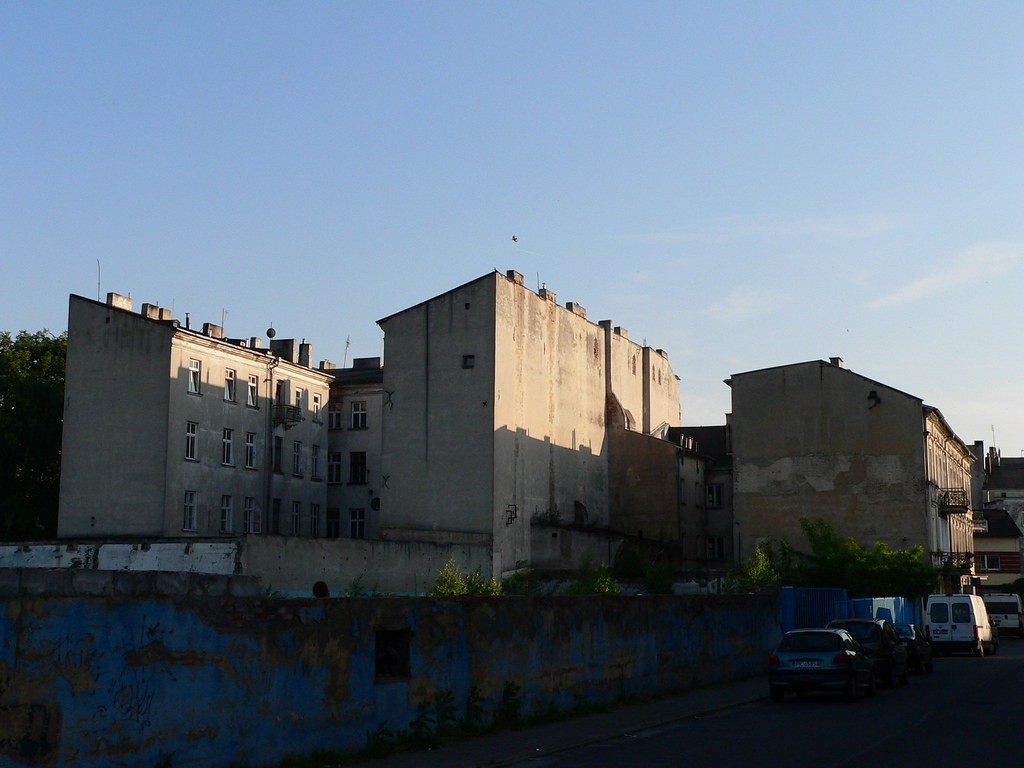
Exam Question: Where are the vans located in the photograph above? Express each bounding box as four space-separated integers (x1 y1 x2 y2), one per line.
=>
983 593 1024 640
925 593 999 656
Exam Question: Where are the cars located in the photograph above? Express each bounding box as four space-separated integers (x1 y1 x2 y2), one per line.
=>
893 624 933 676
766 628 874 703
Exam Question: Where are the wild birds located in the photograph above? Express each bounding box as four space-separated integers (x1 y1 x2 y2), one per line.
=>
512 234 518 242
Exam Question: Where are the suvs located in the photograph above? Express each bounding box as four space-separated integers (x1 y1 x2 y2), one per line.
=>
824 618 911 689
